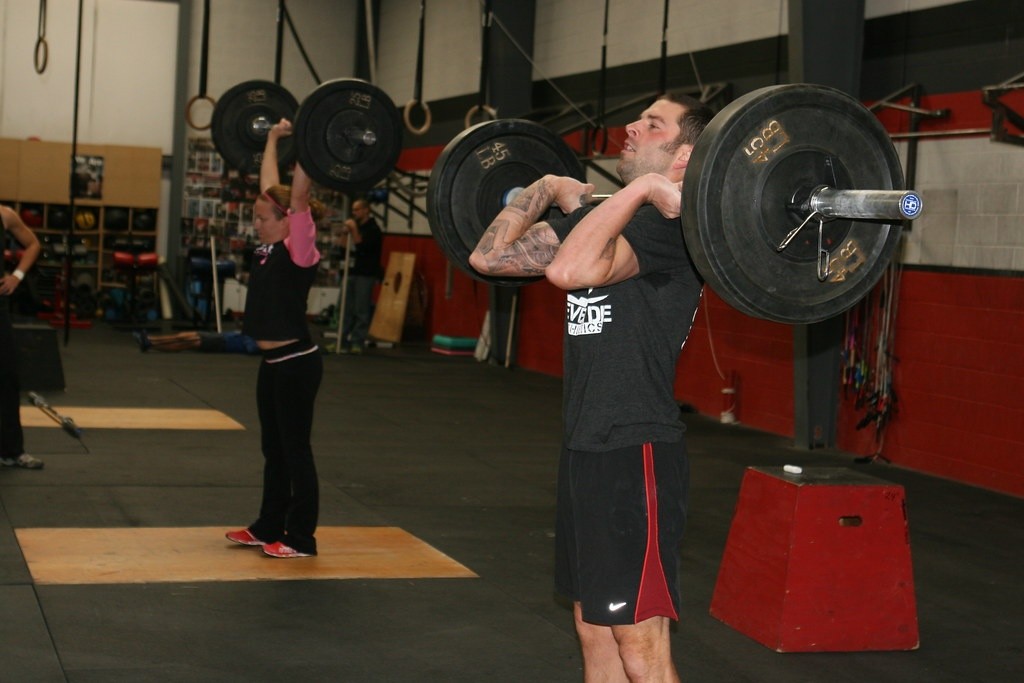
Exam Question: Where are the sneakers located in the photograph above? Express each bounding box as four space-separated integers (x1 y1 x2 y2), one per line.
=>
226 529 267 547
263 541 310 557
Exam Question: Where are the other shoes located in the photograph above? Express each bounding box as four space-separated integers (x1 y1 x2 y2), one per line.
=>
1 453 44 468
323 341 350 354
351 339 362 355
140 329 149 351
133 332 141 345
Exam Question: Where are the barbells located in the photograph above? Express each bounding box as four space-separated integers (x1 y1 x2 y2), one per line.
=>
210 78 405 193
425 82 924 326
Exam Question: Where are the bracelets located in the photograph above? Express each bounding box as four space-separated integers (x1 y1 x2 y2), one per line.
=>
13 270 23 279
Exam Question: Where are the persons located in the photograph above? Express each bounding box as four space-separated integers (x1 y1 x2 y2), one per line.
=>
337 200 384 346
470 91 715 683
0 206 43 469
132 330 260 354
225 119 323 557
